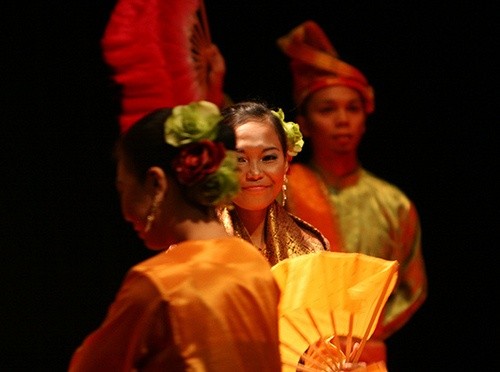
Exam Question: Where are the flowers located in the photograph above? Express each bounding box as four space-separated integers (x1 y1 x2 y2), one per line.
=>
165 100 241 207
270 108 305 175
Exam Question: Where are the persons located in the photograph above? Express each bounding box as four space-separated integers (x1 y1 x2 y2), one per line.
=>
204 22 426 372
214 102 368 372
68 98 283 372
